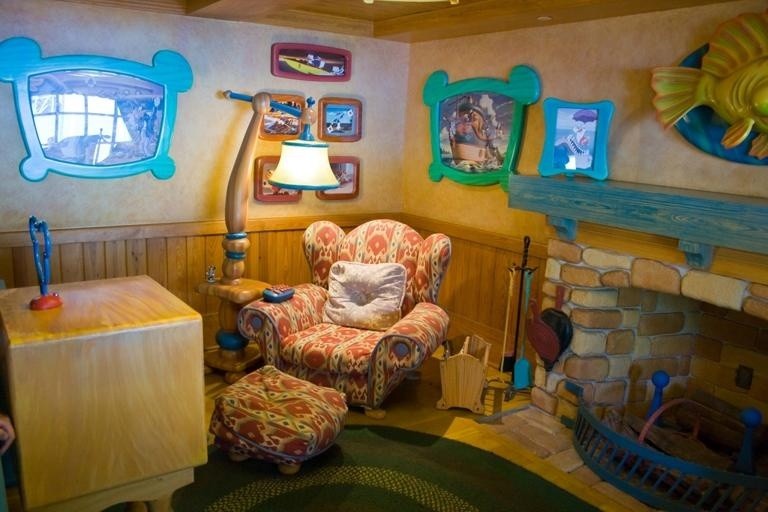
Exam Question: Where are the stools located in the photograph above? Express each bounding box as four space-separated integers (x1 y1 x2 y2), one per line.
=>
211 365 348 473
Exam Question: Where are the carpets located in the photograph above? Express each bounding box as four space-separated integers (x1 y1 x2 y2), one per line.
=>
171 424 603 510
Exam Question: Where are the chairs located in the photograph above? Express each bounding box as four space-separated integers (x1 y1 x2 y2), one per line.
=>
236 217 454 420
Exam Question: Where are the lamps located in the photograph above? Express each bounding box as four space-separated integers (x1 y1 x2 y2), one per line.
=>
194 91 342 385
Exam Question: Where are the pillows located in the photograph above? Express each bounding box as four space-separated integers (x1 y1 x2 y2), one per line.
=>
321 261 408 330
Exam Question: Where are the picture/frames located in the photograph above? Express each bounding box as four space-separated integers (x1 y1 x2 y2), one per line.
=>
537 95 616 182
317 96 363 143
258 93 306 140
272 41 352 82
421 65 542 192
317 155 360 201
254 155 303 203
0 36 194 182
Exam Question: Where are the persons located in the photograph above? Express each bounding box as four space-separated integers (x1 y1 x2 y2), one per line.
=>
0 413 17 453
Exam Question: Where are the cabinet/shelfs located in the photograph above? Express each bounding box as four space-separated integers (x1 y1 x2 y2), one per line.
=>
4 274 208 512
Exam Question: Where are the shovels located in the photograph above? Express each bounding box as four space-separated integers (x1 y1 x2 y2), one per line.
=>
513 269 532 391
